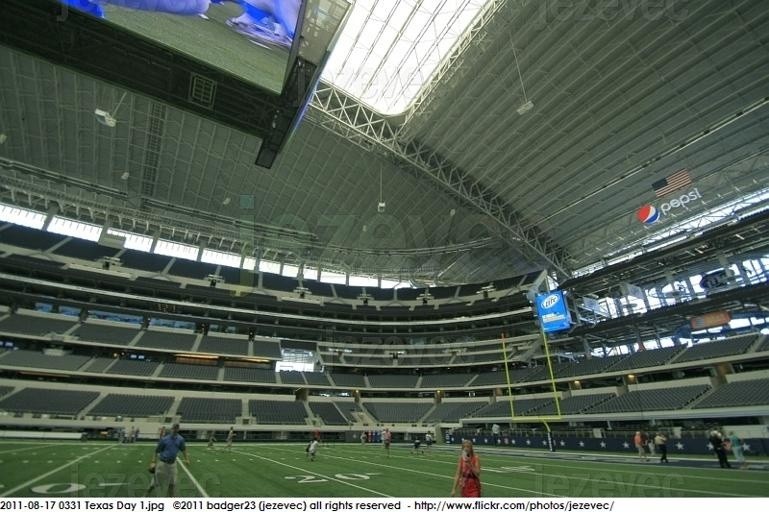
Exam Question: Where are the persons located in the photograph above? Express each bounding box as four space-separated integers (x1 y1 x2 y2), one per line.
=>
118 423 769 497
